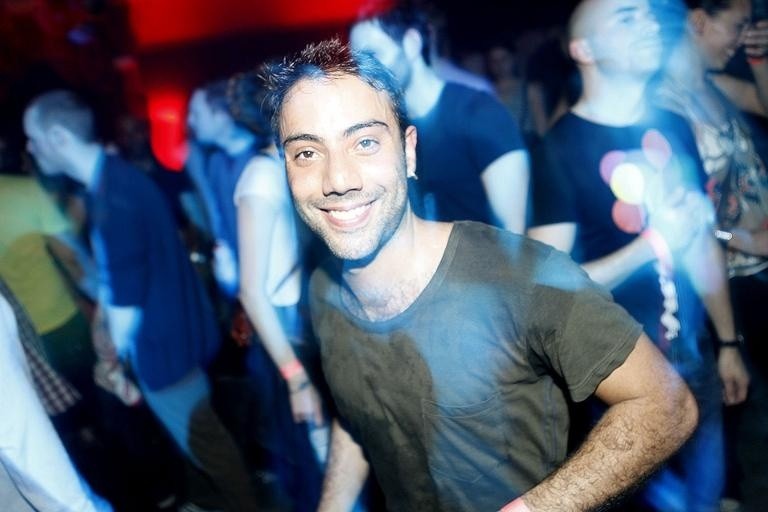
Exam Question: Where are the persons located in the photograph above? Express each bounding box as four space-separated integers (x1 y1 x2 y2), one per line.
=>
0 0 767 512
